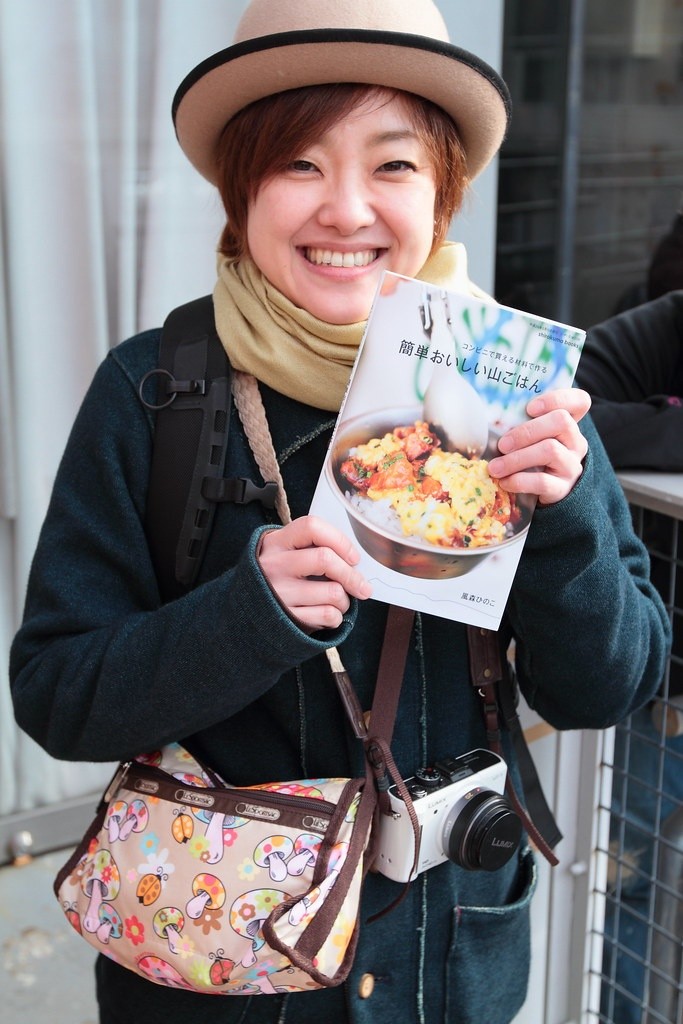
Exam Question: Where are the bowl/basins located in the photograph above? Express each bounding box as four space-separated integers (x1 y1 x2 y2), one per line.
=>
323 407 535 580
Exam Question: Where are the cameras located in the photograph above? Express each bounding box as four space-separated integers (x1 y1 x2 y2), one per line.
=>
374 749 523 883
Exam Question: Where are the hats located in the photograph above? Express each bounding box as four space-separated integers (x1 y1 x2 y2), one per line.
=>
173 0 510 185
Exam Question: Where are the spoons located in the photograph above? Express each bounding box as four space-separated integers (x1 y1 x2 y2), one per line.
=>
422 287 489 458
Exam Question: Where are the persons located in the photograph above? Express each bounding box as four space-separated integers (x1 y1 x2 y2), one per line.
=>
576 290 683 1024
10 0 669 1024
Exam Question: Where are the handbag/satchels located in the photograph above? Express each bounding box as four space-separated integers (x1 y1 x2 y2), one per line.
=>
53 745 376 994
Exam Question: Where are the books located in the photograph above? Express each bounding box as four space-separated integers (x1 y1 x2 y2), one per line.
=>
307 270 587 631
